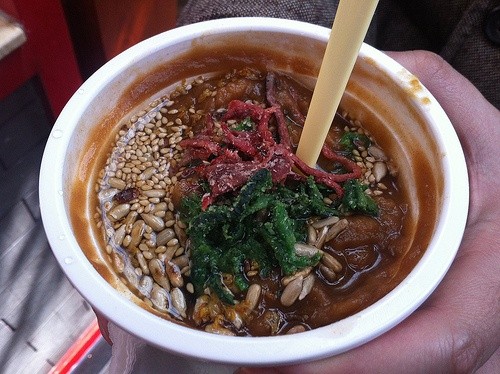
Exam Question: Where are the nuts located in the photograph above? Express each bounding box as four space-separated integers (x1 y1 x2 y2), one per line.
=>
94 91 399 337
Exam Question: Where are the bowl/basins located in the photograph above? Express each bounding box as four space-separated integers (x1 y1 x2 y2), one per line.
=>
38 14 472 367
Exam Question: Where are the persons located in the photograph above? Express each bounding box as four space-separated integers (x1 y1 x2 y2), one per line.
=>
86 41 500 373
